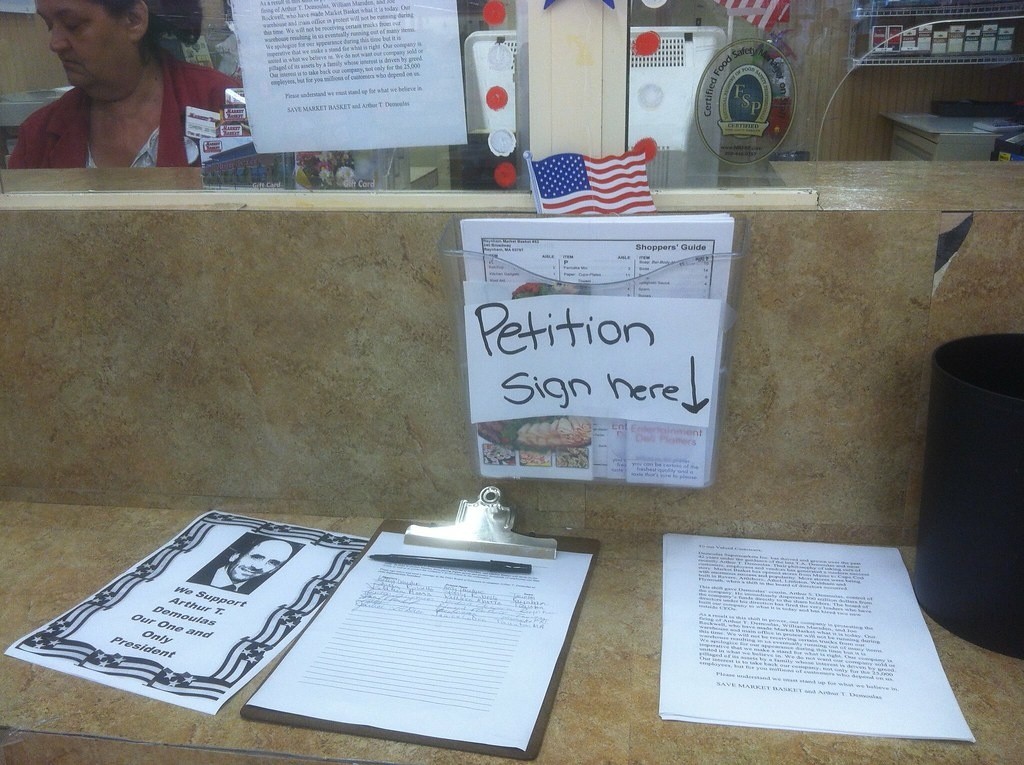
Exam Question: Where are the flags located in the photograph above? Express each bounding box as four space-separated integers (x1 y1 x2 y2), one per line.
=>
531 148 658 215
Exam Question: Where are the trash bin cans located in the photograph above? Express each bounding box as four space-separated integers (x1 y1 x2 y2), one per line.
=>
902 333 1024 664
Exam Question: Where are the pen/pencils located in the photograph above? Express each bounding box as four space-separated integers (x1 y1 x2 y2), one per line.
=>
368 553 532 573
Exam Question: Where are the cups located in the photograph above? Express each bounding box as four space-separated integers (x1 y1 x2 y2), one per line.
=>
913 333 1024 659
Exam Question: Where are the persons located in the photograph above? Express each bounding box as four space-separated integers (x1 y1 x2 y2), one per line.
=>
190 540 294 595
7 0 254 169
